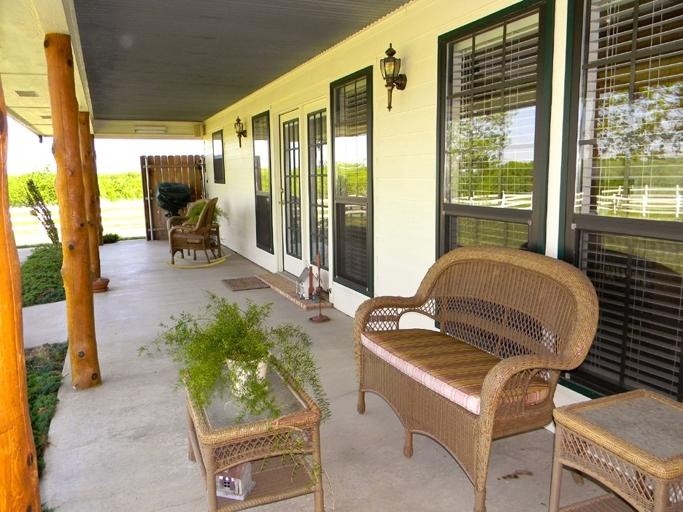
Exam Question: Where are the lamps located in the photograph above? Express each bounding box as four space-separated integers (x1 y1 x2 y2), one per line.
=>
379 41 408 112
233 115 247 149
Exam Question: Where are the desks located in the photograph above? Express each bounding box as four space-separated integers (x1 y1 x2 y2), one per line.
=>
547 388 683 512
181 357 325 512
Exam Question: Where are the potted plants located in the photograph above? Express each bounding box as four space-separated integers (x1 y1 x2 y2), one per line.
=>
134 289 333 491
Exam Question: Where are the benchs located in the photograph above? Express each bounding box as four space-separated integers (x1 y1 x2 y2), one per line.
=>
353 245 600 512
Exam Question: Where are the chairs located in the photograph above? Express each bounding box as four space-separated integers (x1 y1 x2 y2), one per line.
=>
166 199 210 255
168 197 217 265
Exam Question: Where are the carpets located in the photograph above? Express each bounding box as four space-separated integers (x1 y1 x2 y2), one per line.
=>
220 276 271 291
